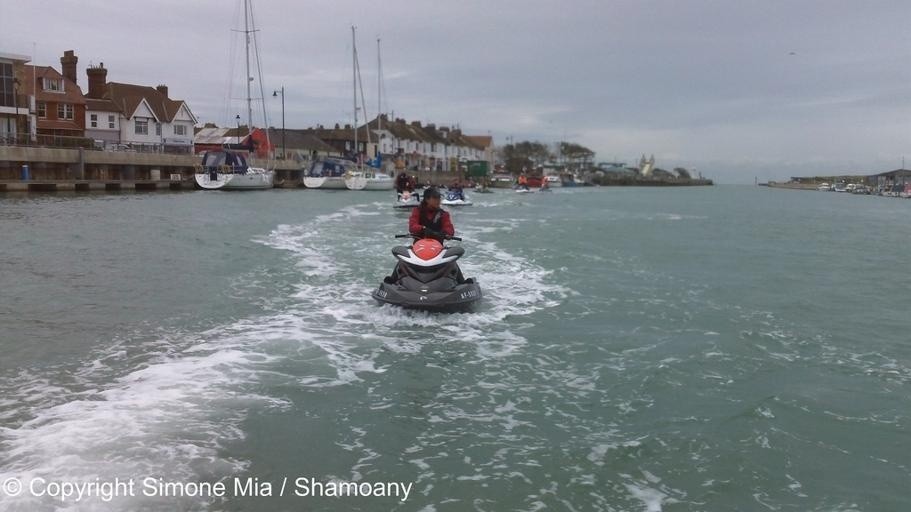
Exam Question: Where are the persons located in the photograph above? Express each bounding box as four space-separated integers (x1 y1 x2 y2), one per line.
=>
393 173 416 200
449 182 464 200
541 177 549 189
518 173 528 185
408 186 455 247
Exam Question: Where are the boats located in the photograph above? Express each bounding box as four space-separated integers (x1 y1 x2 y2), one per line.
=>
422 183 448 194
473 184 552 195
394 191 424 207
372 231 481 308
441 190 472 206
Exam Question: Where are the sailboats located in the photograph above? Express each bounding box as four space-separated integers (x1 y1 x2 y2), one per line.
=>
194 1 279 190
303 23 376 188
344 39 396 191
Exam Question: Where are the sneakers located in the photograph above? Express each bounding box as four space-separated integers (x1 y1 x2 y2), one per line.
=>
384 276 398 284
462 278 474 284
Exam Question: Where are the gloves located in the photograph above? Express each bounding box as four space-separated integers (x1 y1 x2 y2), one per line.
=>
424 227 434 236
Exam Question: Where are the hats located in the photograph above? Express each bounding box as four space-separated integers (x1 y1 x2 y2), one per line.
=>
424 187 446 199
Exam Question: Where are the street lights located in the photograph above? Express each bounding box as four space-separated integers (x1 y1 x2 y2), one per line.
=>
236 115 241 149
12 77 22 145
